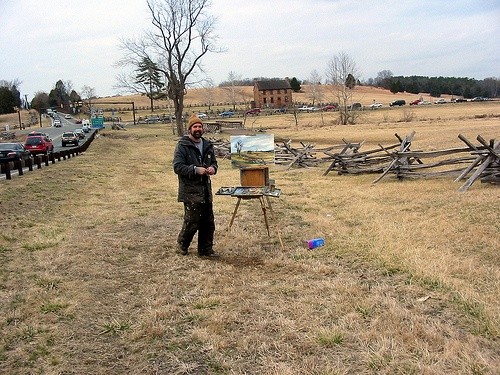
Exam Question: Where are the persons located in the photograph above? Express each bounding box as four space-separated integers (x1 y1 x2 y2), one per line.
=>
172 113 220 258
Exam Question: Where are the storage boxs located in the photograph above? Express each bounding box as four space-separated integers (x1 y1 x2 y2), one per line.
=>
239 167 269 187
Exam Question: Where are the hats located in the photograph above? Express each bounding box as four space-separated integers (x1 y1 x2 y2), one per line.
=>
187 114 203 130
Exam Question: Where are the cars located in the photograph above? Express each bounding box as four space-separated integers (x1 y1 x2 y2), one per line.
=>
0 142 34 164
145 111 209 124
297 102 385 113
219 110 235 118
73 128 85 139
45 108 62 128
75 118 92 133
64 113 72 119
409 97 485 106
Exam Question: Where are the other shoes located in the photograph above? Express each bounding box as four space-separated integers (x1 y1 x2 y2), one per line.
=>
180 244 188 255
203 252 220 258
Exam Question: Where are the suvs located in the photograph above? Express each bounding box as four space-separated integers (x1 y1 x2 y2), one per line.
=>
245 107 262 115
22 131 54 157
61 131 79 147
389 99 406 107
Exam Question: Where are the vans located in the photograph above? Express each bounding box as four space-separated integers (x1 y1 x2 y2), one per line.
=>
274 109 287 114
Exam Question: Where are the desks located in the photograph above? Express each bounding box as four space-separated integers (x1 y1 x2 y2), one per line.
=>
215 184 283 239
